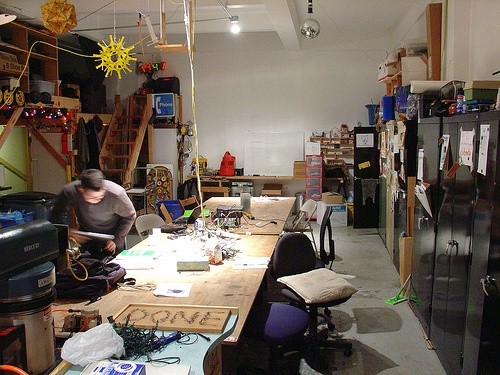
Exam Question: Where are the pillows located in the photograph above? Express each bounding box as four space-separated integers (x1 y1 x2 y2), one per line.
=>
276 268 358 303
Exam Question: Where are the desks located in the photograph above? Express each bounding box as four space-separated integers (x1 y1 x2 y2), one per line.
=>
126 188 149 234
53 197 296 375
187 175 306 181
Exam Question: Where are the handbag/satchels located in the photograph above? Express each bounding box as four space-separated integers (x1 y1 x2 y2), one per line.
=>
55 258 126 299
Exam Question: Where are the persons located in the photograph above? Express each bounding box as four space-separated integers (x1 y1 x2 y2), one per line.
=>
52 169 136 262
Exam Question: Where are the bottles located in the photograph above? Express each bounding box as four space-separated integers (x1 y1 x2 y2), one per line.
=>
80 308 102 332
240 185 251 212
457 93 463 115
75 310 82 333
63 309 75 332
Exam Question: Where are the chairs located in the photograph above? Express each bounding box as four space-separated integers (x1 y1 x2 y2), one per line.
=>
283 199 318 256
179 195 199 211
273 233 352 371
238 301 309 375
135 213 165 241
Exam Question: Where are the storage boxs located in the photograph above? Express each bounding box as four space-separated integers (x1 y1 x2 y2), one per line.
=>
29 80 56 95
305 141 323 221
463 80 500 100
419 92 439 118
293 161 306 177
316 192 348 228
377 60 397 82
261 183 283 196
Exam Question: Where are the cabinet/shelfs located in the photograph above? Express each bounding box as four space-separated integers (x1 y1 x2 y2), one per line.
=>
0 22 58 106
351 110 500 375
